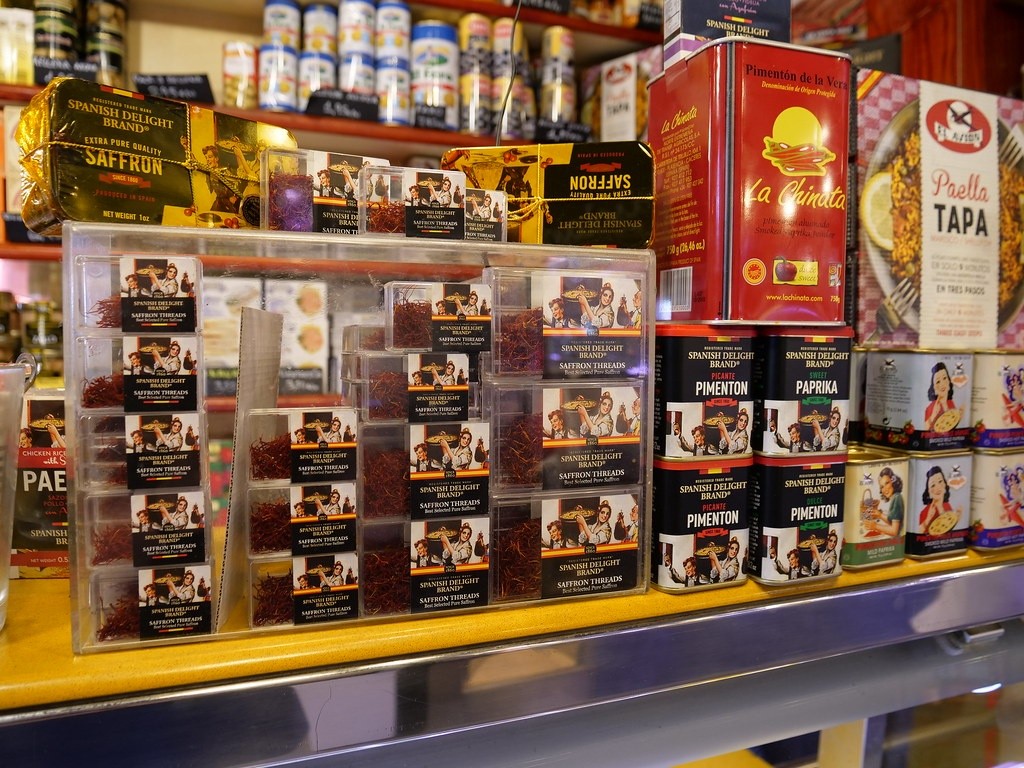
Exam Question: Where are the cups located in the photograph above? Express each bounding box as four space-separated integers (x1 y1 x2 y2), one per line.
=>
0 352 38 632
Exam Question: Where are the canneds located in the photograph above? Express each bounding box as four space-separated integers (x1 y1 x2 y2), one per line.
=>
32 0 129 93
651 323 1024 597
226 0 601 144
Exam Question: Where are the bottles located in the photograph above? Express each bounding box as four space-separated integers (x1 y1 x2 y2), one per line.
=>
0 0 35 87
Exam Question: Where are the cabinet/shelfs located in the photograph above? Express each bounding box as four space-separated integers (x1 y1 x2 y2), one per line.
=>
0 0 1024 768
